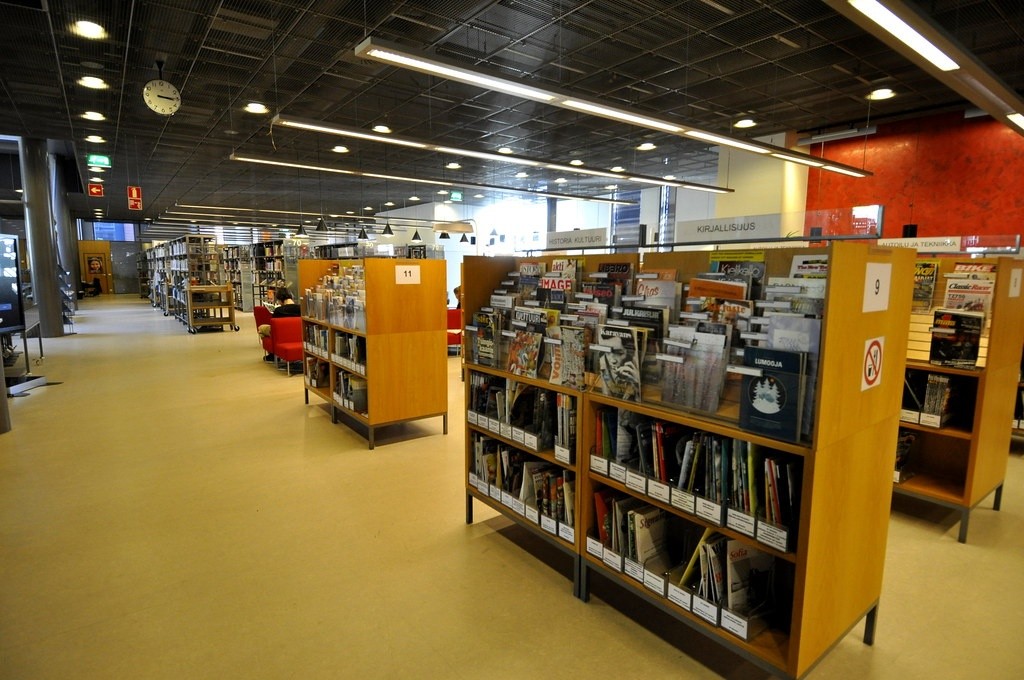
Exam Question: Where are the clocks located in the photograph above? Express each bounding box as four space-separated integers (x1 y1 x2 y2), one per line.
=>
143 80 181 115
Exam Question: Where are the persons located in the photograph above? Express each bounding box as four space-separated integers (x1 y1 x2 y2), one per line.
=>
88 257 103 274
262 286 302 363
598 328 639 403
453 285 461 310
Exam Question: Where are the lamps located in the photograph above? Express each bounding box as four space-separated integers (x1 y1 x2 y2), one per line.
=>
137 0 1024 248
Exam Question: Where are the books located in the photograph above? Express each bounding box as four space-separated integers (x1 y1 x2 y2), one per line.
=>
896 428 919 471
469 261 824 614
909 261 995 369
925 373 953 415
302 275 365 412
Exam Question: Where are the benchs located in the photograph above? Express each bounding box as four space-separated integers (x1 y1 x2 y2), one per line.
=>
447 309 461 357
254 304 303 376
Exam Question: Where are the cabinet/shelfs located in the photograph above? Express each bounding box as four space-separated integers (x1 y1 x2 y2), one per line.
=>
892 256 1024 543
455 239 918 680
297 255 450 450
142 234 444 336
